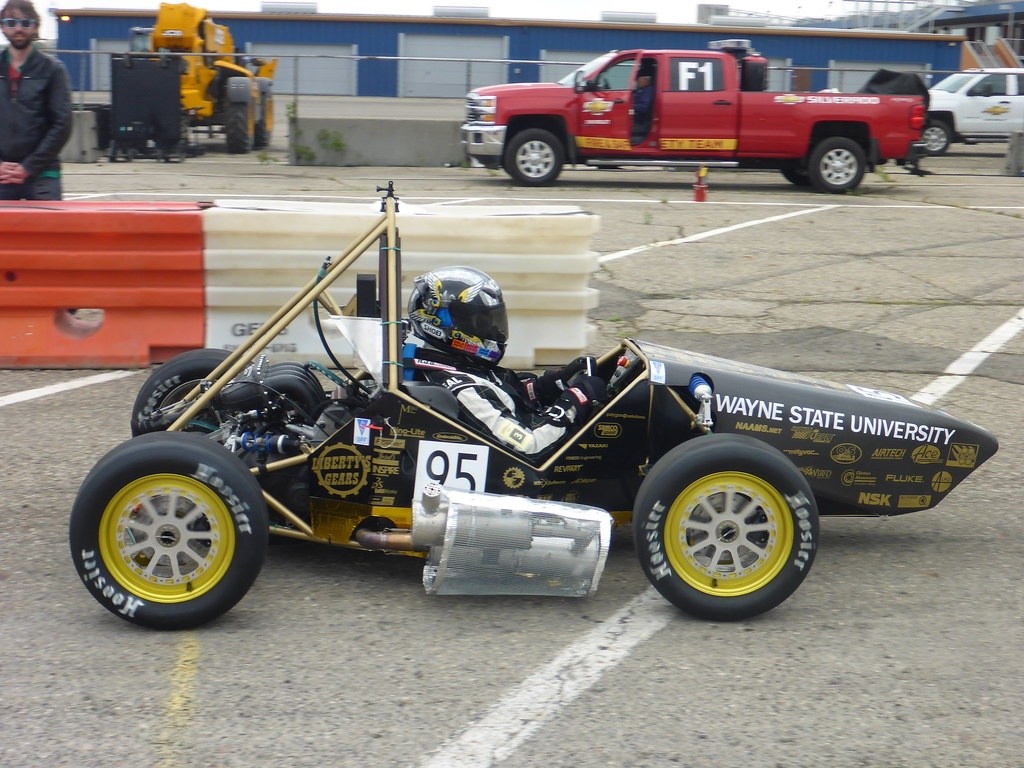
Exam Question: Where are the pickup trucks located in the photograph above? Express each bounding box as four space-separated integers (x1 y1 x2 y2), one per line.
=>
458 30 934 196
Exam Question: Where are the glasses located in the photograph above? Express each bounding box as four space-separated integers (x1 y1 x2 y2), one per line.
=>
0 18 35 29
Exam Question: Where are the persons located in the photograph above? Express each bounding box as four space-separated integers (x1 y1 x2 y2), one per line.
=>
632 64 656 124
408 266 606 466
0 0 76 316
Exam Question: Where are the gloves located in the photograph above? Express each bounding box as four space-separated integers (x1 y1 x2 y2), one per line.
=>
538 356 596 393
544 374 606 430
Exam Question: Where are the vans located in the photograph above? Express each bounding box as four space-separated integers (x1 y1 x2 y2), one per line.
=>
919 68 1024 158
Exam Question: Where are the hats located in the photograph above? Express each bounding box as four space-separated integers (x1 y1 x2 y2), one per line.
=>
636 66 654 80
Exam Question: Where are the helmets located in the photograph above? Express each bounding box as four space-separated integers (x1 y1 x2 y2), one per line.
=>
407 266 509 373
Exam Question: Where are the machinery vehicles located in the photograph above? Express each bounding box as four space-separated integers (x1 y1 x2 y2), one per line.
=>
125 4 278 153
